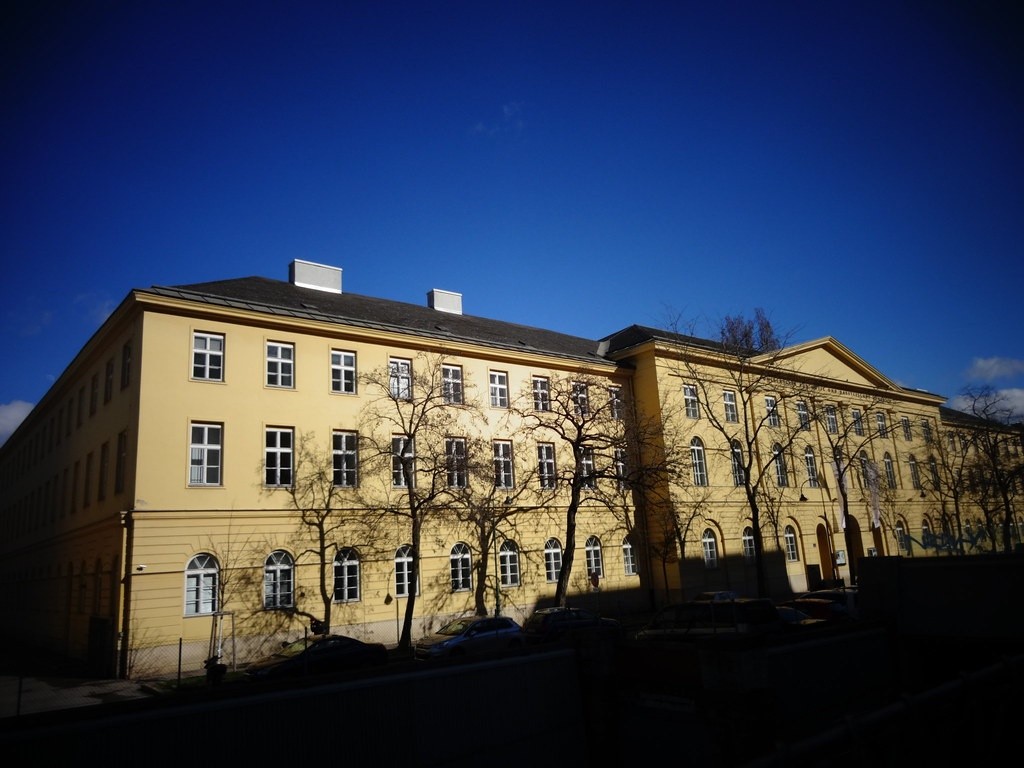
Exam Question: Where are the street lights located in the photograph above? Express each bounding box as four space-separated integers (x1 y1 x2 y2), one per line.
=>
920 479 952 555
491 479 515 618
799 479 840 584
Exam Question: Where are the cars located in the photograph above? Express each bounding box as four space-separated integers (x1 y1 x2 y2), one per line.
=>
630 586 863 659
522 606 624 656
414 614 530 667
241 634 389 690
